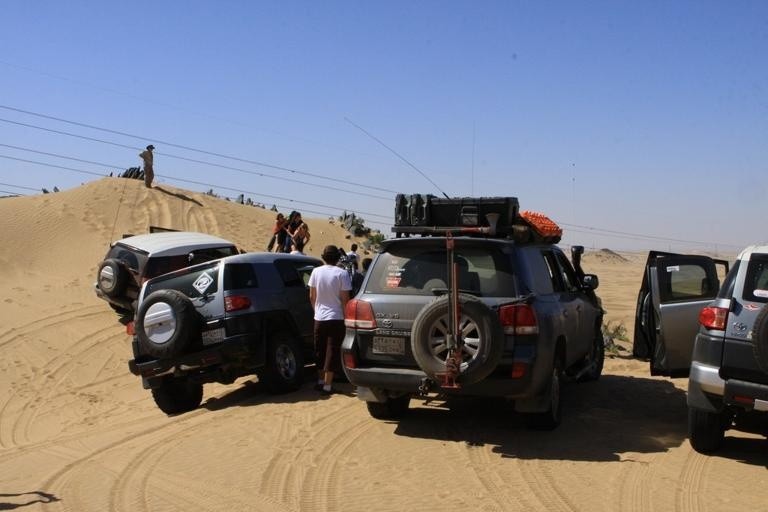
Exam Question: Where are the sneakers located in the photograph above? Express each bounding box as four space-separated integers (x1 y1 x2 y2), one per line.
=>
314 384 335 396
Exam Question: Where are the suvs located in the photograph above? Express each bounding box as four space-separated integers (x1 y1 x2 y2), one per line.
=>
125 250 364 414
340 192 607 429
632 242 768 453
94 226 238 313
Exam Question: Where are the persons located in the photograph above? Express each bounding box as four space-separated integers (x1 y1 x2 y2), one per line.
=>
336 243 373 294
139 144 155 188
307 246 353 397
267 211 310 253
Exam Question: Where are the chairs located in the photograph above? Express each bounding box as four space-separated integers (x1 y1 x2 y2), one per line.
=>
456 257 480 291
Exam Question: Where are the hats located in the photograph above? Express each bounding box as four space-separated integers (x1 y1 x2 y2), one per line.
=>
146 145 154 149
325 245 338 256
